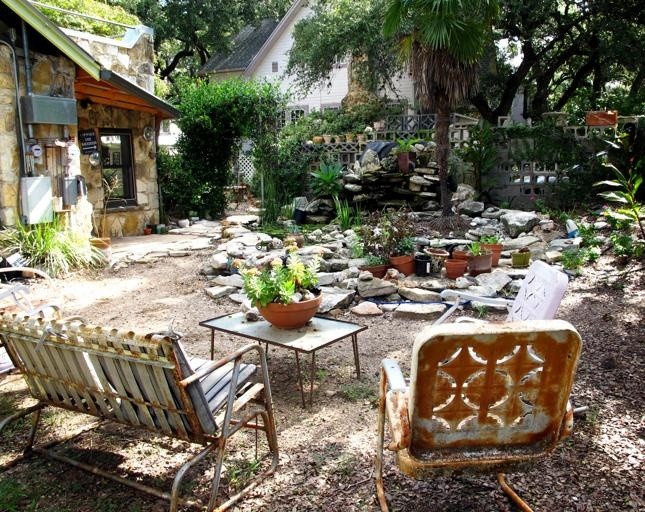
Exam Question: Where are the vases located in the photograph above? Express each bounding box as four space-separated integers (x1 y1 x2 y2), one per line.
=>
146 224 157 234
143 228 152 235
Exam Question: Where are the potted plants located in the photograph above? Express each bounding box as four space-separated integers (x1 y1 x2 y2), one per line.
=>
511 247 532 268
231 238 325 329
286 223 304 248
358 211 504 280
312 128 365 144
396 137 425 174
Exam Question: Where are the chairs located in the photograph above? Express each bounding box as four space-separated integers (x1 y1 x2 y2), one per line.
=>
372 318 584 512
432 259 570 362
1 283 278 511
